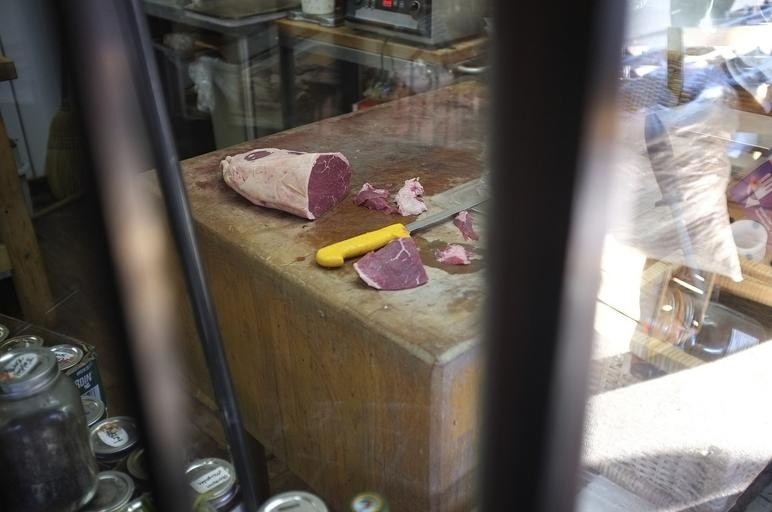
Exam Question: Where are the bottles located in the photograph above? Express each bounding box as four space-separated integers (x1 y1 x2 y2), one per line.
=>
0 346 99 511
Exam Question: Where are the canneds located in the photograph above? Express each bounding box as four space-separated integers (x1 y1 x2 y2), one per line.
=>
0 325 154 511
186 458 326 510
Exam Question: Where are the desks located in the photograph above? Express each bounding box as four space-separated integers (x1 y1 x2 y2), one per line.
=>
139 80 491 507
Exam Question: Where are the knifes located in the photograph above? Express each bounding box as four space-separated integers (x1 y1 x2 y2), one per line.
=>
313 192 491 270
